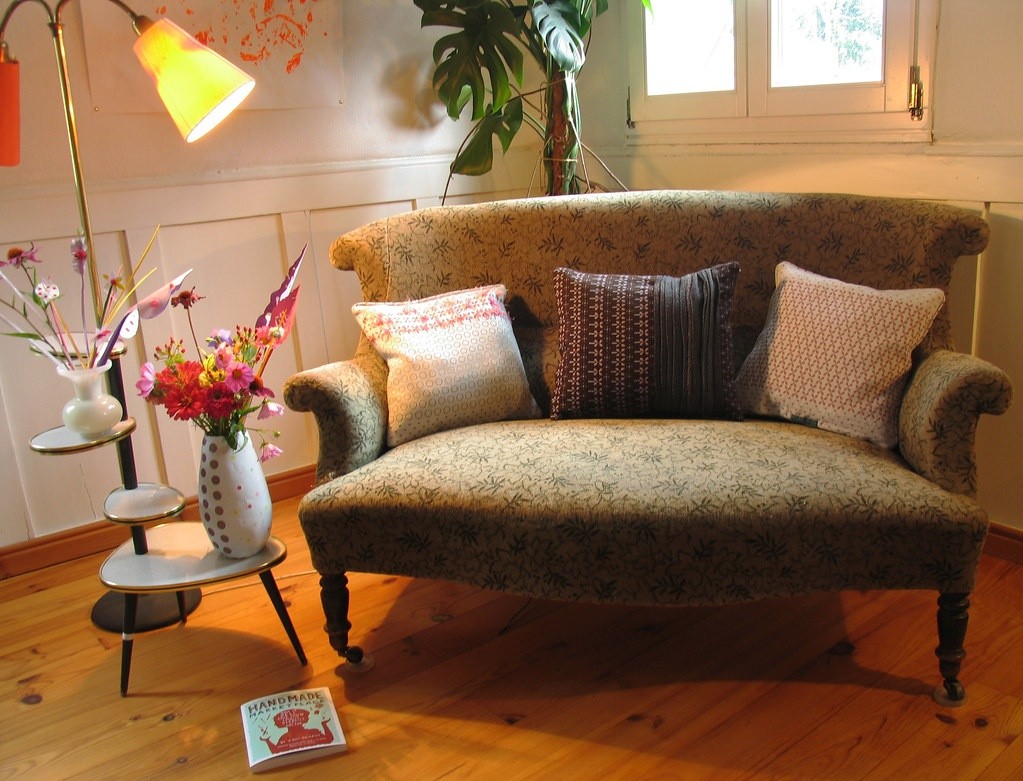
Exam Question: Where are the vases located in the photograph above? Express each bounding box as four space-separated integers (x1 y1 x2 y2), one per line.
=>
56 349 120 440
196 427 273 562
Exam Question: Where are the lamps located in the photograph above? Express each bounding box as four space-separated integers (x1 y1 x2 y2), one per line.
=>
1 2 253 633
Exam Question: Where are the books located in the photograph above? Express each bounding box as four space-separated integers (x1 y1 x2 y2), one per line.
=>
240 687 348 774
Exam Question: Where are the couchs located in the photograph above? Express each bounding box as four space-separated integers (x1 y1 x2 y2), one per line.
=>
281 190 1013 698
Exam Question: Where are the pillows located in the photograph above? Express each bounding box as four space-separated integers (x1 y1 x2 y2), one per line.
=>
357 274 543 453
554 259 744 422
732 262 947 444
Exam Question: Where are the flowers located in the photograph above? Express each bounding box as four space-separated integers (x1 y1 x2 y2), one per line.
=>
1 232 182 382
134 256 311 457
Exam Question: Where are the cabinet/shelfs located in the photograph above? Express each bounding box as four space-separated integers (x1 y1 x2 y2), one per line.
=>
27 412 310 696
